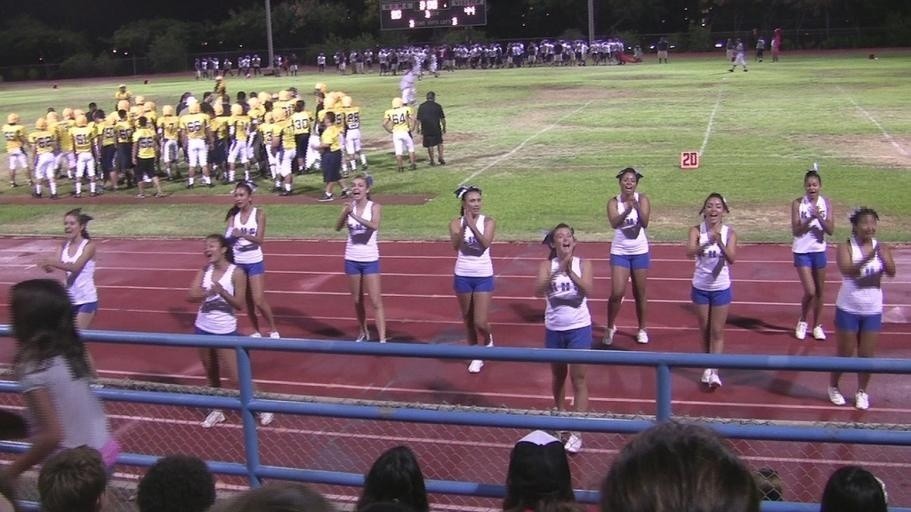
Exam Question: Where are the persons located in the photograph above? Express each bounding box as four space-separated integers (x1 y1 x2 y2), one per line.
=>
334 175 386 343
602 167 651 345
185 234 275 429
36 208 101 379
503 420 887 511
193 37 670 80
535 223 592 453
791 171 835 340
449 185 494 374
1 278 430 512
2 69 447 201
726 26 782 72
223 180 280 339
686 193 737 388
827 206 896 409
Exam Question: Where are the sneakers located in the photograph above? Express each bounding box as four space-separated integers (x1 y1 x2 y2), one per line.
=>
10 164 251 199
700 368 724 391
258 412 274 425
399 158 445 172
854 390 868 410
467 358 484 373
564 432 582 453
250 331 263 337
200 410 226 428
795 319 807 340
270 331 280 339
827 385 845 406
812 326 826 340
637 329 648 344
602 324 616 346
484 333 493 348
269 163 368 202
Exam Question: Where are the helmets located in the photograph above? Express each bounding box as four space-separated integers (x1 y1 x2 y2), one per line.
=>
8 113 19 124
35 108 86 130
314 83 351 108
117 90 292 123
392 97 403 109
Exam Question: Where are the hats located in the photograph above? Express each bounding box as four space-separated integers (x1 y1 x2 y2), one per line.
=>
508 428 568 486
